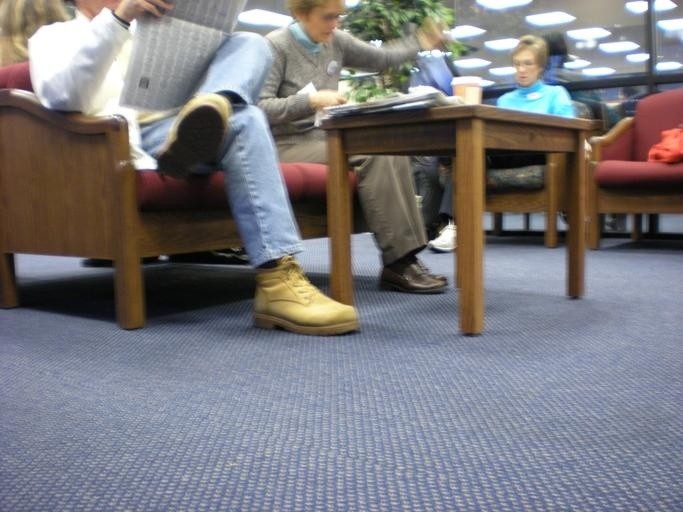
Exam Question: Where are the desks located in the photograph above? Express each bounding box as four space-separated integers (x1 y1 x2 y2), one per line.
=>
319 104 593 335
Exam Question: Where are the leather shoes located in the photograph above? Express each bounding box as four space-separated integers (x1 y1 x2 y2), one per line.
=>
382 257 448 293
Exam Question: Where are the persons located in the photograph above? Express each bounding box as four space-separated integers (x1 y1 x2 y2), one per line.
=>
255 2 449 297
617 85 641 103
429 34 593 254
26 1 356 337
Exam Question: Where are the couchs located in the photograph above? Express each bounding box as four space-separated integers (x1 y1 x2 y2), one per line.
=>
0 62 371 331
589 86 683 250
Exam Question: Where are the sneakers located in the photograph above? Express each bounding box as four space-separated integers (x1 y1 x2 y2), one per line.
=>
427 223 457 252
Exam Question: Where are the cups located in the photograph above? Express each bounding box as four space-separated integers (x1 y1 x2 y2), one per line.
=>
449 75 482 106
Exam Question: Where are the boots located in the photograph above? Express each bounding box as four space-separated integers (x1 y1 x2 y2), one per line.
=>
158 94 229 179
255 255 358 336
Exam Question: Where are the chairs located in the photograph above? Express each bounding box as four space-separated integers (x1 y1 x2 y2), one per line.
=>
484 100 590 248
